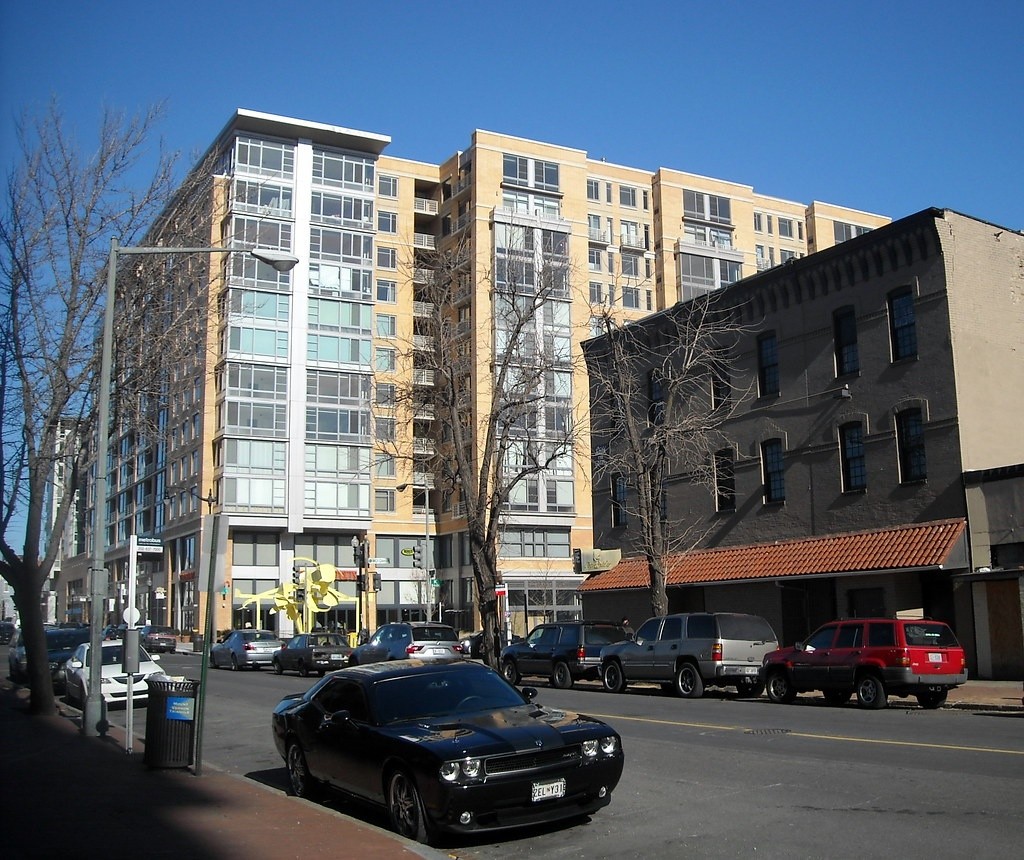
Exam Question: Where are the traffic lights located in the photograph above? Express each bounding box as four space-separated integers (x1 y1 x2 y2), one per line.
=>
292 565 300 585
295 588 305 602
413 544 425 570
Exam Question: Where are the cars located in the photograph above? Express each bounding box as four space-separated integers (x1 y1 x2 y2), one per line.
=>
271 658 625 846
65 639 167 713
460 629 525 659
101 623 178 655
270 632 355 678
209 628 288 672
0 617 91 689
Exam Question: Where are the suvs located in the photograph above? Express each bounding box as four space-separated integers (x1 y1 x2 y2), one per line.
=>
348 619 465 662
499 619 629 690
597 609 780 699
757 615 968 710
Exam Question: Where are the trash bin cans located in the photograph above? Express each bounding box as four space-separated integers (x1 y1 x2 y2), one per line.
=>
193 635 203 652
143 676 200 769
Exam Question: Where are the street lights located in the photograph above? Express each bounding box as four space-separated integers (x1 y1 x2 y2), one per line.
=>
145 576 154 626
351 536 371 634
397 475 433 622
85 236 300 739
